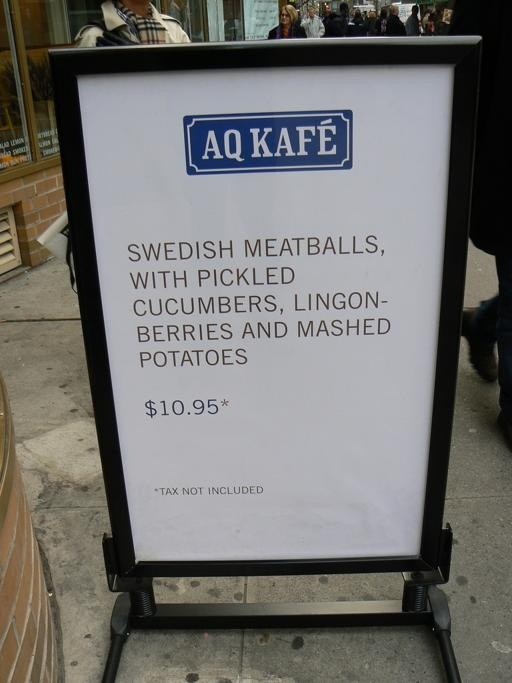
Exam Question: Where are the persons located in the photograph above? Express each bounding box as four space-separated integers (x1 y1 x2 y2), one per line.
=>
169 0 447 39
449 0 511 450
74 0 192 47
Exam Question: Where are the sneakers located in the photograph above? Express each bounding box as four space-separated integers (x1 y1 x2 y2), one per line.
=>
460 308 498 382
